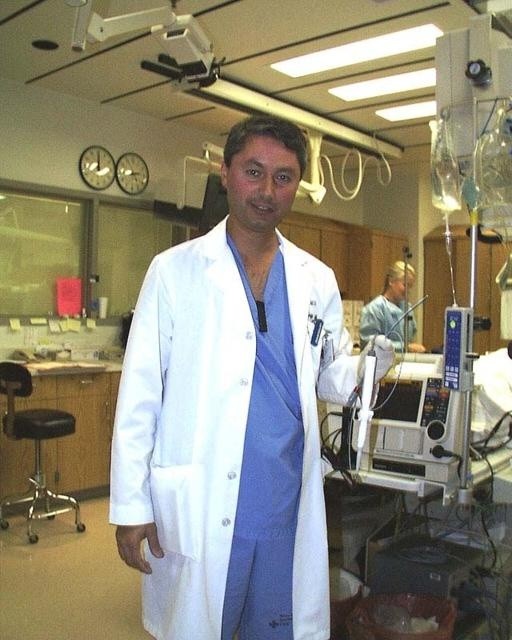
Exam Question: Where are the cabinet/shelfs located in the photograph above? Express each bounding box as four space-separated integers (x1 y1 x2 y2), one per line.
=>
0 373 118 507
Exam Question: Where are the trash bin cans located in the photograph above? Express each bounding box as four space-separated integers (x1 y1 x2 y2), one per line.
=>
359 594 456 640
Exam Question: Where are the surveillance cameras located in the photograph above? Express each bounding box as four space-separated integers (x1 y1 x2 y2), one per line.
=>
158 19 220 86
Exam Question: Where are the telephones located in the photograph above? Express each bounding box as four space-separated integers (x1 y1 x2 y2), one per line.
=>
14 348 42 364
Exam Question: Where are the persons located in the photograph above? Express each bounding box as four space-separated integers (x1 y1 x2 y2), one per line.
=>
108 113 394 640
359 261 426 353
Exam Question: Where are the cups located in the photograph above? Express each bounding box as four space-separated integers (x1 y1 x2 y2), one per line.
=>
98 297 107 317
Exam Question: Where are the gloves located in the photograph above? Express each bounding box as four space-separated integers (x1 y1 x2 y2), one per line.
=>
359 334 394 385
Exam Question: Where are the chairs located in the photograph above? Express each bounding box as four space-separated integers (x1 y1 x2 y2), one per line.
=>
0 361 84 544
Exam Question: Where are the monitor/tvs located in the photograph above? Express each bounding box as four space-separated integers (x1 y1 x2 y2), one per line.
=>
369 380 425 424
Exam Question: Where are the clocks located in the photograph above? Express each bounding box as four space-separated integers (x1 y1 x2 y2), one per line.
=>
79 146 150 196
